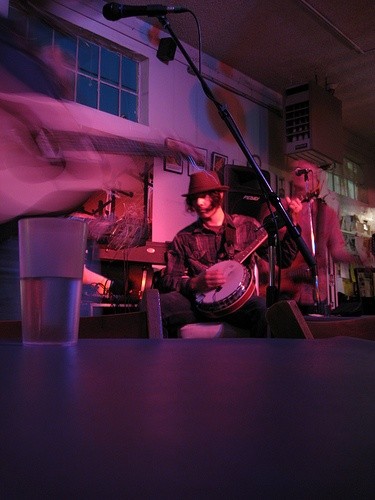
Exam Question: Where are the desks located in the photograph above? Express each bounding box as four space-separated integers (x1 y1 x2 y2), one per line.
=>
0 339 375 500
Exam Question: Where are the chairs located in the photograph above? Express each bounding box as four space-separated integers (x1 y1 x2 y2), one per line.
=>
0 288 165 342
265 299 375 340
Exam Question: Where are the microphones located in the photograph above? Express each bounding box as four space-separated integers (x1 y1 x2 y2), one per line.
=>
103 2 187 21
295 168 311 176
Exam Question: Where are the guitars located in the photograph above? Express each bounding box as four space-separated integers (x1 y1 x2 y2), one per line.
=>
192 188 319 319
0 90 205 224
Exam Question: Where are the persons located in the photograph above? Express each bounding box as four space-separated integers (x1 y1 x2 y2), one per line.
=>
255 159 375 316
69 212 112 294
136 170 303 338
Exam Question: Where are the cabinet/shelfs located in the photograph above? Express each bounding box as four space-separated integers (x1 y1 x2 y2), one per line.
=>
284 79 344 167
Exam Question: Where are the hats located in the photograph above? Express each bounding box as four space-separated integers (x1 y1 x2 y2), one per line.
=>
181 171 229 197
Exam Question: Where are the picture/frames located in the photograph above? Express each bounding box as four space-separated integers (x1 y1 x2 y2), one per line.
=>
162 137 184 174
187 147 209 176
211 151 228 184
